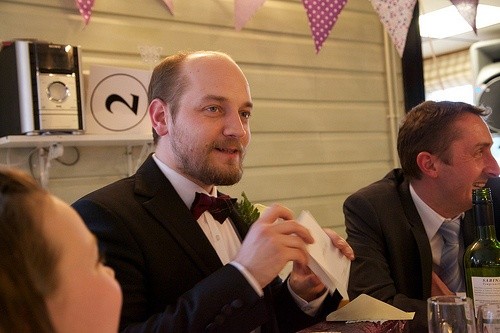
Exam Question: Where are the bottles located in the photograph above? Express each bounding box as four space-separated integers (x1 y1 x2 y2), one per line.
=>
464 187 500 318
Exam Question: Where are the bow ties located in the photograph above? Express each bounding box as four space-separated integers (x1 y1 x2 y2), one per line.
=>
190 192 237 224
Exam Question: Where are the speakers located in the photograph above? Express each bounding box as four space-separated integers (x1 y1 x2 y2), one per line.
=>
471 39 500 176
0 39 85 137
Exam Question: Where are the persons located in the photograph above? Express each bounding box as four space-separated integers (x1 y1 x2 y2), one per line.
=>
342 99 500 333
70 48 355 333
0 161 122 333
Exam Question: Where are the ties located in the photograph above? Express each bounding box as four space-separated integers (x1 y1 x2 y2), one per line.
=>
438 219 465 294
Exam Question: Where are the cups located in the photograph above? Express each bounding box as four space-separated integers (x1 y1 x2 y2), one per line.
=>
477 303 500 333
427 296 477 333
